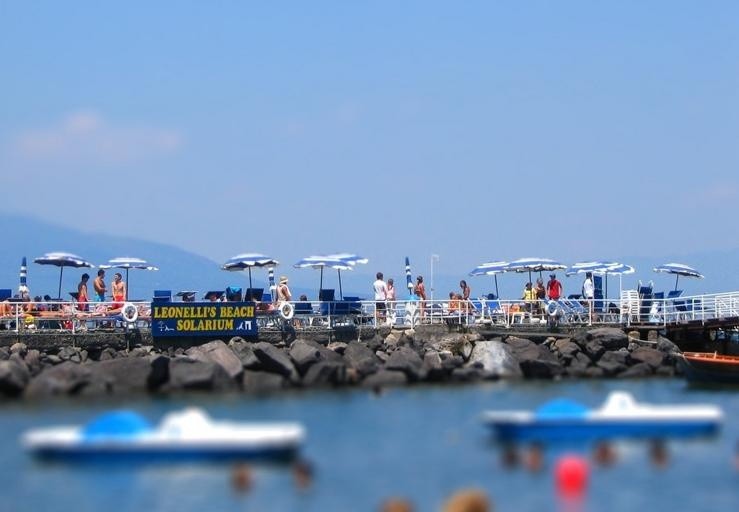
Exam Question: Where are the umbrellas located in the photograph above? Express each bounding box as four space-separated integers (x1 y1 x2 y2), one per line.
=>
33 251 97 297
531 256 566 278
568 259 616 299
219 251 279 302
468 260 520 299
17 256 33 299
294 255 351 303
590 263 634 301
506 255 569 278
99 256 157 302
322 251 370 299
653 261 704 311
403 257 421 298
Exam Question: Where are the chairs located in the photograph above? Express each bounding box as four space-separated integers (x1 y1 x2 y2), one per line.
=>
469 296 524 325
620 279 718 326
537 295 594 326
153 285 373 323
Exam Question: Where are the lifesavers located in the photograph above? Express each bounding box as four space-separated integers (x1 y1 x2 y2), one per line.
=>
121 304 138 322
280 303 295 320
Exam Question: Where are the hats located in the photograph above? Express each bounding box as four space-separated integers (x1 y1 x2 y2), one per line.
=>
549 273 555 277
279 276 287 283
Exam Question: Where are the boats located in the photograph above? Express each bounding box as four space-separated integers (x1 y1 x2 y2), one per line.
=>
22 405 305 466
477 390 725 445
665 348 739 386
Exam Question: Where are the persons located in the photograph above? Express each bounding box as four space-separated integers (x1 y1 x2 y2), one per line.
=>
373 272 397 326
276 275 292 302
414 276 428 320
77 270 126 314
182 293 222 302
448 280 470 327
1 294 73 329
584 271 594 307
300 295 308 302
522 273 561 315
